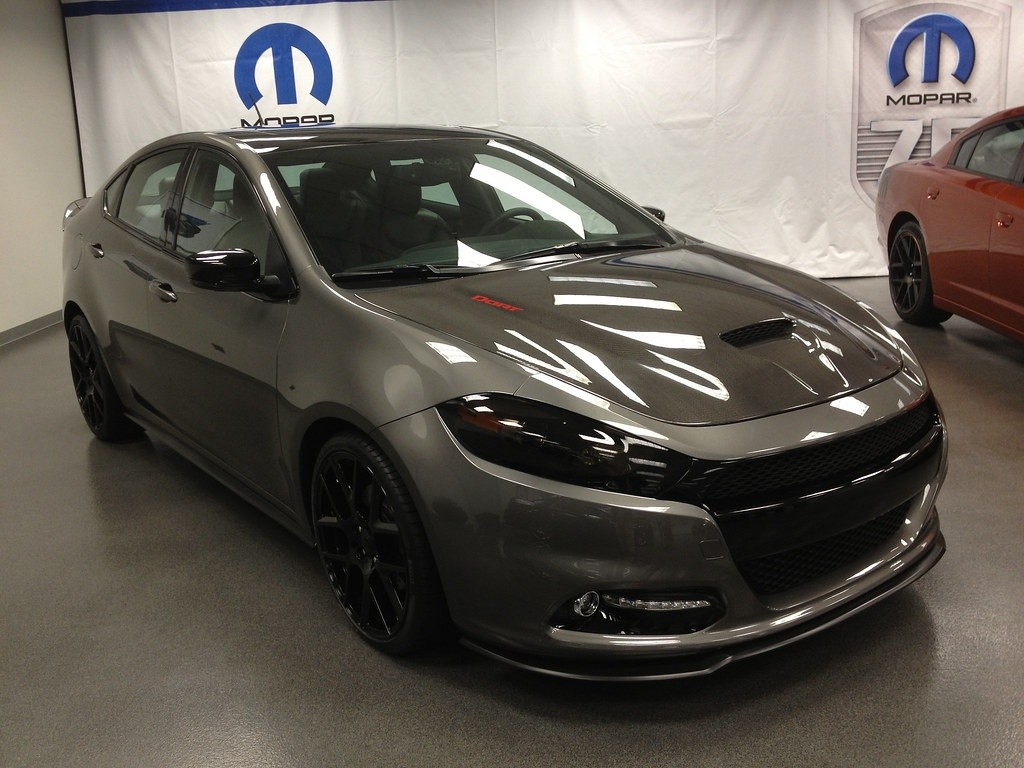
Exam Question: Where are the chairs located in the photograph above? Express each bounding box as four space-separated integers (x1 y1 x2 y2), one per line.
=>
155 164 469 277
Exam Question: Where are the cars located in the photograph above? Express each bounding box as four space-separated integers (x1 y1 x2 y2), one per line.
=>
877 103 1024 347
62 121 954 691
